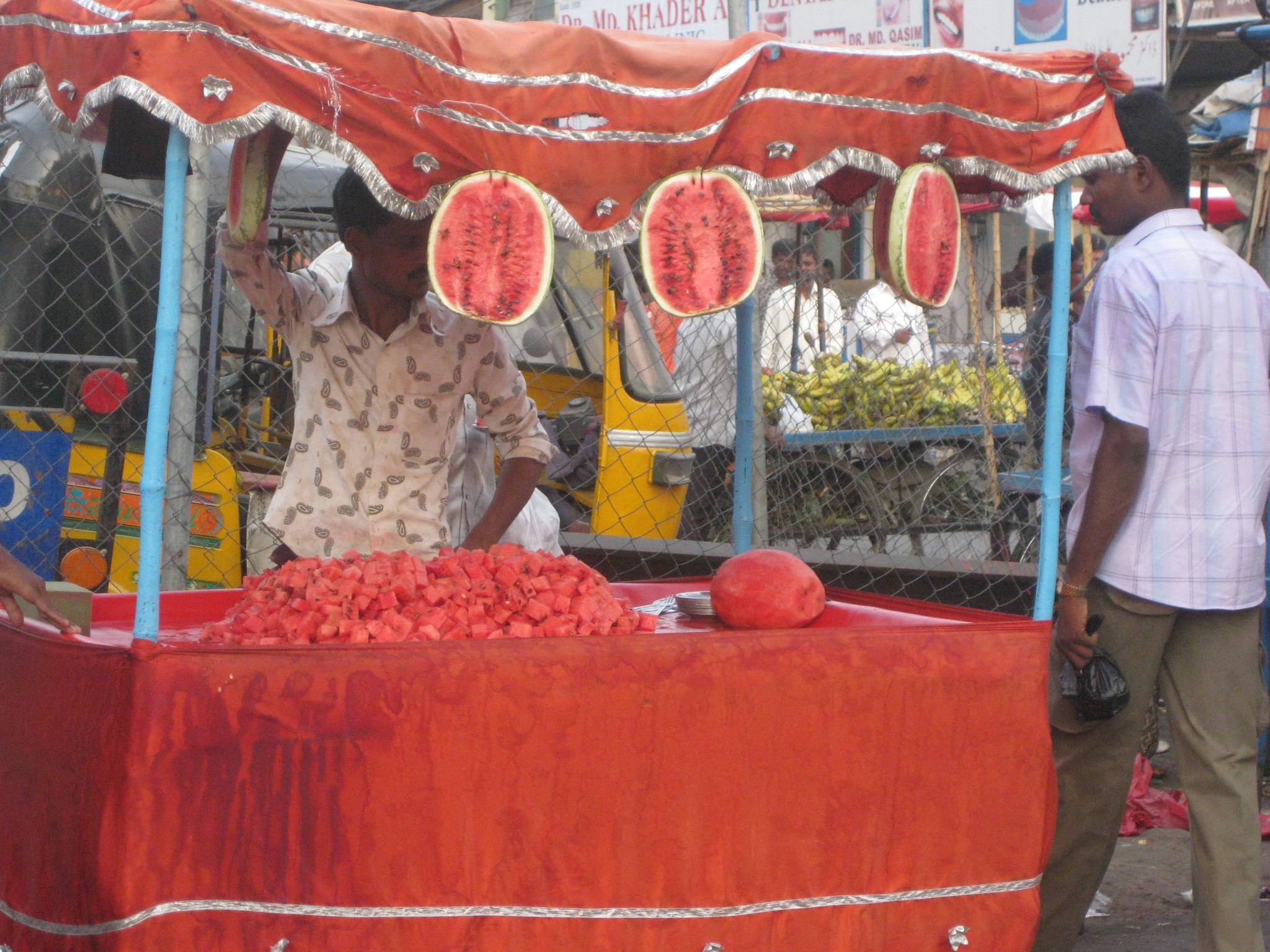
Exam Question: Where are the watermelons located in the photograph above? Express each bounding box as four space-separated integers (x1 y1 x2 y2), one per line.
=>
888 162 961 309
710 549 826 629
197 541 658 645
427 169 554 326
227 123 268 248
638 169 764 317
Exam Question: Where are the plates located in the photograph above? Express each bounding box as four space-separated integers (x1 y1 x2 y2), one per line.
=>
675 591 717 618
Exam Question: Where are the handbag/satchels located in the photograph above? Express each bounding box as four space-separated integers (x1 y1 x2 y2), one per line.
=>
1058 612 1132 722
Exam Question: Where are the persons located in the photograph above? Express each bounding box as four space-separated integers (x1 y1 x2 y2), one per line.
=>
759 243 845 375
820 257 834 284
852 275 934 369
0 546 81 635
216 119 567 569
758 237 799 337
1033 89 1269 952
668 304 784 543
984 233 1109 467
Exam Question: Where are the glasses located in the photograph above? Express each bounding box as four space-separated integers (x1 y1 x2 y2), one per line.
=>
1070 265 1084 275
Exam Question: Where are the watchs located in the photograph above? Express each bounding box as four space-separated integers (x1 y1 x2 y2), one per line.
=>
1056 573 1090 598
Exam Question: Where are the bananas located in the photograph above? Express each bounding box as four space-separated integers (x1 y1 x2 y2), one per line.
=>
760 350 1028 431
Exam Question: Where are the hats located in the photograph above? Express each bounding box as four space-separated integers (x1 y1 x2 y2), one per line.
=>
1031 241 1084 276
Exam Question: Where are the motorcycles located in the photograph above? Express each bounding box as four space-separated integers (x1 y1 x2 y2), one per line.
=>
0 91 694 580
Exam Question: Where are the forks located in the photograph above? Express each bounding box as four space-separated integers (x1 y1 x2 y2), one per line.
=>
631 594 677 615
